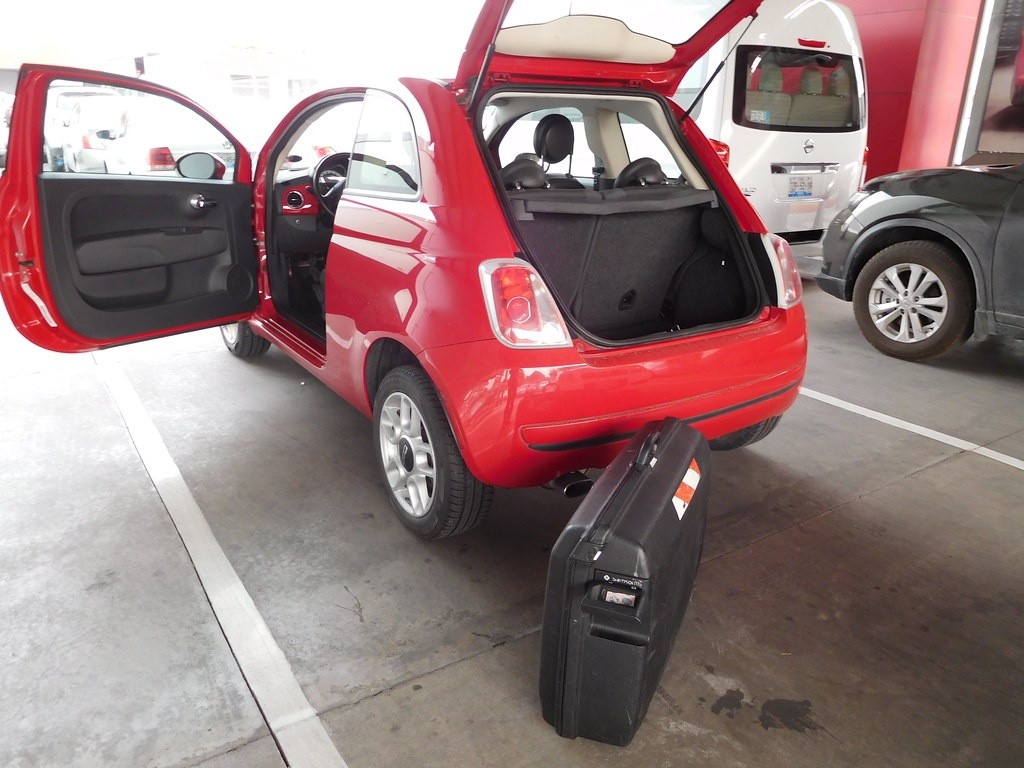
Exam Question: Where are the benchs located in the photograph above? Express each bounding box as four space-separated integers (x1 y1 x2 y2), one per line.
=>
746 64 792 126
787 67 849 127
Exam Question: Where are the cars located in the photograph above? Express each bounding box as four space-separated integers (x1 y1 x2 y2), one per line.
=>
0 1 810 543
812 159 1023 366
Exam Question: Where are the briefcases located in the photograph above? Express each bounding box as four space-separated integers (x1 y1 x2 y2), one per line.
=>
539 415 711 749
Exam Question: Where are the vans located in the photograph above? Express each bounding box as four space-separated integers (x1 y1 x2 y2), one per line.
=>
419 0 869 250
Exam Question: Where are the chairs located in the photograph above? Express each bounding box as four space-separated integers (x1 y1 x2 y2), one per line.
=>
496 158 549 192
614 157 668 190
534 113 584 192
